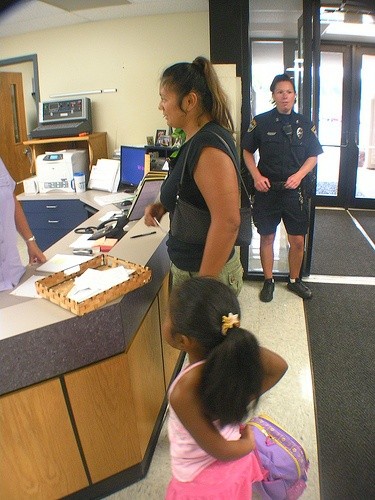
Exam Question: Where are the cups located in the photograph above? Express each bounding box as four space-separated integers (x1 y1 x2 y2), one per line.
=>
71 172 87 194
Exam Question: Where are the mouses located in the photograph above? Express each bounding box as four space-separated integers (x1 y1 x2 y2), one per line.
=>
121 201 133 206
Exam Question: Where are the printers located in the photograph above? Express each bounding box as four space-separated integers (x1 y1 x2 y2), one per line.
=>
36 148 89 194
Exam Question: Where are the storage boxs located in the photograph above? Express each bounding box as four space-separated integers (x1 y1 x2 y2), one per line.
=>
35 254 152 316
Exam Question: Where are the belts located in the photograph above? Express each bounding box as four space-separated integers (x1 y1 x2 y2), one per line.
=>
270 182 289 192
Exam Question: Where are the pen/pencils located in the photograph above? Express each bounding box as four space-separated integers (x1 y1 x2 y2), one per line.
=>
130 231 156 238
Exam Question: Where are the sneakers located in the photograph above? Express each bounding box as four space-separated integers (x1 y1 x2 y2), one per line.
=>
287 274 312 298
259 282 275 302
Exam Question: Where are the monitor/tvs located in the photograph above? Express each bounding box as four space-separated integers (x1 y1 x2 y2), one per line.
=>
121 146 145 193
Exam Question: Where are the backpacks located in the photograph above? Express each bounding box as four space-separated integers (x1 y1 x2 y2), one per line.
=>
245 412 309 500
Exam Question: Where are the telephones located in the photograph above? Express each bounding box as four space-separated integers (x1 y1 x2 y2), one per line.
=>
96 215 129 240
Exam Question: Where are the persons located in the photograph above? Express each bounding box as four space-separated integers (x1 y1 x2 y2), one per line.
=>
240 75 323 302
143 56 245 296
0 157 45 293
163 277 289 500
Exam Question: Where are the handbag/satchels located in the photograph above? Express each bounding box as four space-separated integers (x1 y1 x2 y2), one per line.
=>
170 130 252 247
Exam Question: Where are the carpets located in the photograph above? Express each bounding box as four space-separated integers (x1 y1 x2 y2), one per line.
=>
304 208 375 277
302 281 375 500
348 210 375 244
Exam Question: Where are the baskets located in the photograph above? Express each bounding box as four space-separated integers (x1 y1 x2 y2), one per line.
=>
35 253 151 316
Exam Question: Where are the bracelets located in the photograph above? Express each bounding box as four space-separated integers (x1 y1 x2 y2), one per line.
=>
24 235 36 244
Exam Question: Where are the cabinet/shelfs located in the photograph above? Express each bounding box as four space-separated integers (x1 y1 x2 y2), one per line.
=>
19 199 88 252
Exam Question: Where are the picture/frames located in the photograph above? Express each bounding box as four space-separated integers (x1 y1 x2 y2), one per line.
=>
156 130 166 146
